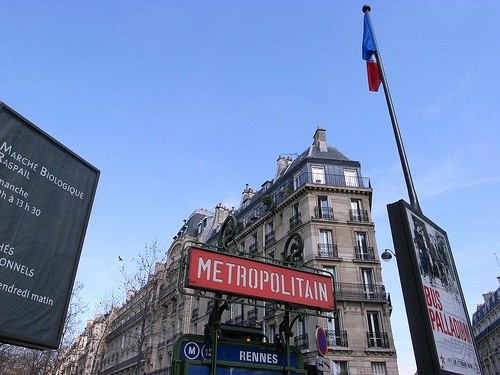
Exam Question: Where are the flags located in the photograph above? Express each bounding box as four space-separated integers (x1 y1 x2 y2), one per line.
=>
361 10 386 92
